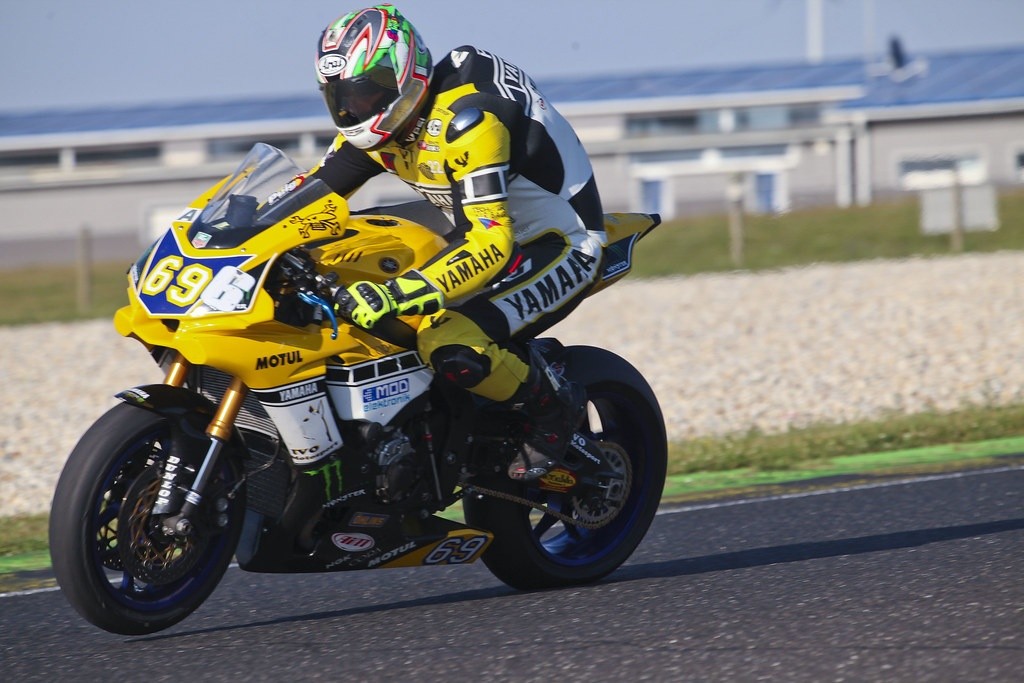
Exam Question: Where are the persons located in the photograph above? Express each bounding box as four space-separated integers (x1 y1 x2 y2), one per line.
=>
208 4 608 479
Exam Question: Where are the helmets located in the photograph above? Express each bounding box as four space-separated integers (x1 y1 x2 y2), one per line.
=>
314 2 434 153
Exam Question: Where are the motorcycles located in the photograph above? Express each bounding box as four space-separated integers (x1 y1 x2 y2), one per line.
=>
49 143 668 638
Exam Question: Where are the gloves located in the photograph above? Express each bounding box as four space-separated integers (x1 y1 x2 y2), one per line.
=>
333 268 444 330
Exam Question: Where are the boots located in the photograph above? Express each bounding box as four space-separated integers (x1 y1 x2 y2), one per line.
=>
507 350 586 482
